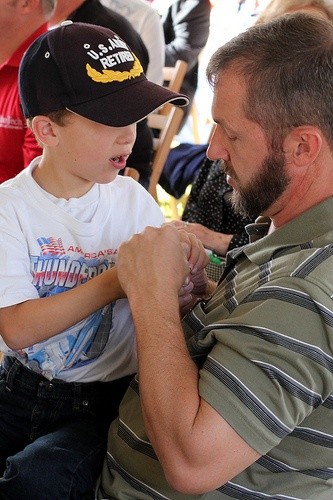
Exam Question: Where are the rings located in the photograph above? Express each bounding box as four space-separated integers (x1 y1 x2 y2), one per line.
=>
184 222 190 227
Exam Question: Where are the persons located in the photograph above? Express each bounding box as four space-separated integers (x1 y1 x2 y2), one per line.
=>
0 20 211 500
94 12 332 500
159 0 332 257
0 0 57 183
48 0 210 190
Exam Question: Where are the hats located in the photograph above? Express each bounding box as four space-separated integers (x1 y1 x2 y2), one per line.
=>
17 22 189 128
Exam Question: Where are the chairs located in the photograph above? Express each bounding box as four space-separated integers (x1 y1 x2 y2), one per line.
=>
147 106 184 203
158 59 187 115
